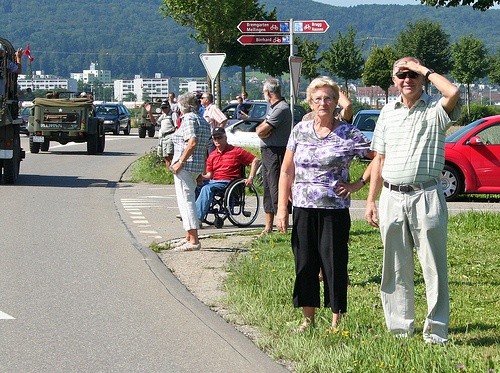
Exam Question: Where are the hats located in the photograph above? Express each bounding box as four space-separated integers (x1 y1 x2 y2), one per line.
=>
160 101 169 107
211 126 224 135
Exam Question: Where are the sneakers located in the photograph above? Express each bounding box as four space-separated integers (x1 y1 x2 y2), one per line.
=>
171 237 188 247
173 242 202 251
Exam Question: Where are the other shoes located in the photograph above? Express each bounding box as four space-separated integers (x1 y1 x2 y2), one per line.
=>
176 214 182 219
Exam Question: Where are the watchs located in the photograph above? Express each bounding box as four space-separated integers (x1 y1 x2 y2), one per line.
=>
425 69 434 79
250 177 253 180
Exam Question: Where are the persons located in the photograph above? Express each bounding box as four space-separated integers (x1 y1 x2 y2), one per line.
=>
274 78 377 337
171 92 211 254
153 90 258 172
364 56 461 348
176 127 262 230
253 77 292 235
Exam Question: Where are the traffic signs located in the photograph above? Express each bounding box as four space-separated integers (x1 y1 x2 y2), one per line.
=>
237 35 290 46
293 19 330 33
236 20 290 33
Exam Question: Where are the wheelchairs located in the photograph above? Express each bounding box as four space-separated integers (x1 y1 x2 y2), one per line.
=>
194 178 261 228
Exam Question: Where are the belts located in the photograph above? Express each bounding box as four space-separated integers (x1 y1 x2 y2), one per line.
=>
382 182 413 192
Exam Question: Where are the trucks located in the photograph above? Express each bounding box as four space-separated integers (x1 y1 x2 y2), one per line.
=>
138 96 170 138
0 38 25 184
27 91 106 153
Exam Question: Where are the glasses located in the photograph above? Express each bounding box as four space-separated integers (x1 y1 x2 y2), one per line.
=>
311 96 336 104
201 97 208 99
396 71 420 79
213 136 223 140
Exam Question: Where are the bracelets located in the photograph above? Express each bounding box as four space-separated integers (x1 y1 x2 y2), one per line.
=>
178 160 186 164
359 178 366 185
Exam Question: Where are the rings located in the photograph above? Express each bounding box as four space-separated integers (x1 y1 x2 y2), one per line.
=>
405 62 407 64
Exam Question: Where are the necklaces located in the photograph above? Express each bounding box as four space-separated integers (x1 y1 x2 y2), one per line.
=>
312 119 334 140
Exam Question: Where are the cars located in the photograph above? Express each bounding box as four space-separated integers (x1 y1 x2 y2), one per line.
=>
439 114 500 202
208 101 308 150
17 106 33 136
94 103 131 135
351 110 381 161
